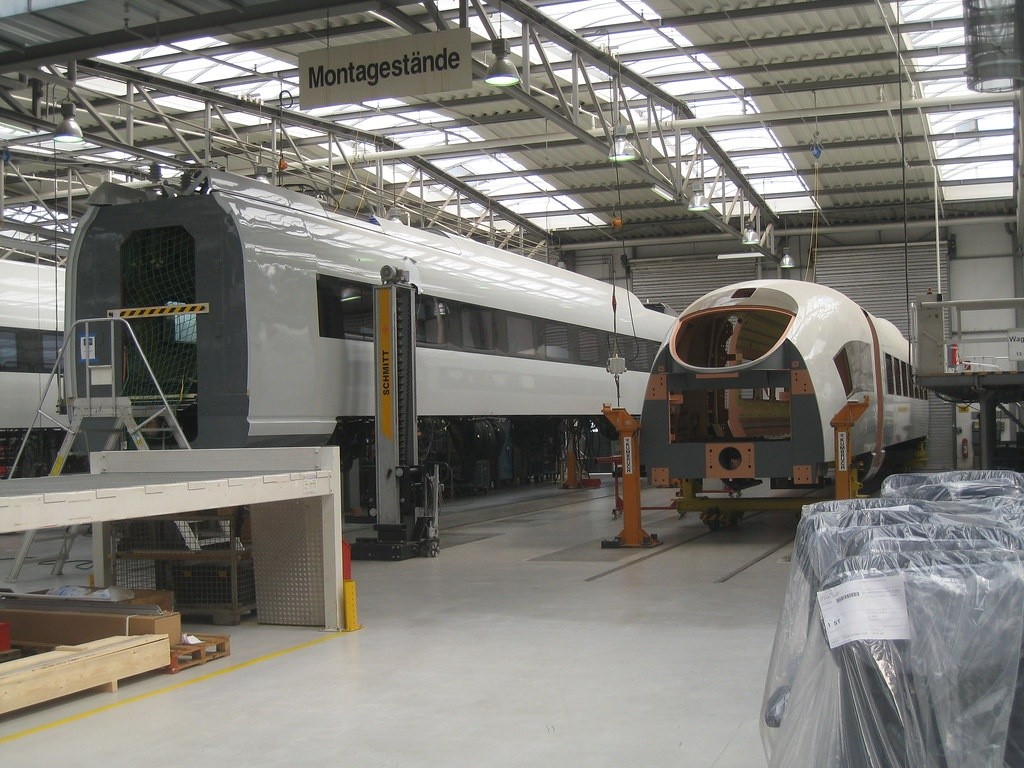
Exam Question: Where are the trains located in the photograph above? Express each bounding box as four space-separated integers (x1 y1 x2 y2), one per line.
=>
0 258 69 429
634 277 932 534
52 164 676 451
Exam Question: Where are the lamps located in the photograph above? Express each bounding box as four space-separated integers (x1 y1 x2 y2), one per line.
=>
779 216 796 268
51 89 84 144
608 76 642 162
741 174 761 245
145 162 164 195
686 102 712 212
482 0 520 86
